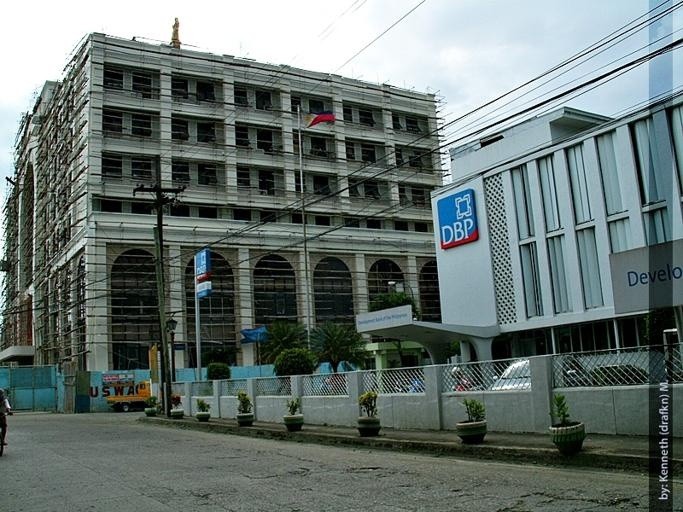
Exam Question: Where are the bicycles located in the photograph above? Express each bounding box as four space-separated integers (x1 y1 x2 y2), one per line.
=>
0 412 12 456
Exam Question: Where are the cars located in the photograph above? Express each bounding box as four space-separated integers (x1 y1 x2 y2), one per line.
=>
489 353 589 390
582 364 650 385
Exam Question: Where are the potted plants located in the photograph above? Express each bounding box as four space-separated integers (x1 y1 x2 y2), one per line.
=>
283 395 304 432
456 398 488 444
196 399 210 422
236 390 254 427
144 396 158 417
549 393 586 456
170 397 184 419
356 390 381 437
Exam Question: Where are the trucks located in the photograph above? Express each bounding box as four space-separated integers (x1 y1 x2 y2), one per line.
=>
106 381 151 412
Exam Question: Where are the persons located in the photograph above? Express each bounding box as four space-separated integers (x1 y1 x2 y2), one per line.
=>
0 388 13 445
172 17 180 42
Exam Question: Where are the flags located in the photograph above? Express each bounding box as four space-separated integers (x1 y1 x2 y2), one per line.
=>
302 113 335 128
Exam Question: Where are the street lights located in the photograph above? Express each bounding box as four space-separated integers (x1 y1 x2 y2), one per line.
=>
388 281 415 317
167 319 177 381
